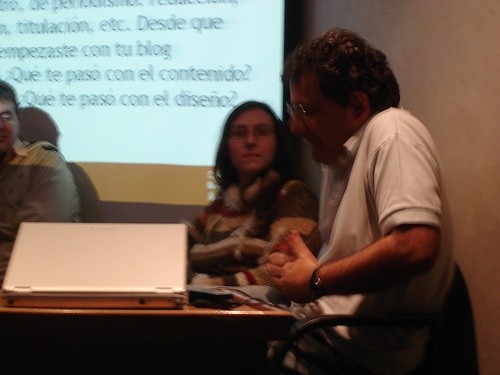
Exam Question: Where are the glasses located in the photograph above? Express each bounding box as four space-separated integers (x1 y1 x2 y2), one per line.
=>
286 97 345 116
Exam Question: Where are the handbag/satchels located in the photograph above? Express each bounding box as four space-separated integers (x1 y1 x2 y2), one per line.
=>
350 170 480 375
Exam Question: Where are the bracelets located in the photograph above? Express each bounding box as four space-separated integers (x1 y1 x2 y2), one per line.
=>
309 266 323 298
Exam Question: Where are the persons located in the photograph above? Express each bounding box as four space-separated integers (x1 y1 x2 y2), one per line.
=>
262 26 457 375
185 101 319 288
0 81 81 289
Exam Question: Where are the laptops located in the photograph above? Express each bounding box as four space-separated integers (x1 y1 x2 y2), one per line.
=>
0 222 187 308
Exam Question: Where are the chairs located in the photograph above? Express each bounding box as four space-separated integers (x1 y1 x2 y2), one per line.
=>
263 259 480 375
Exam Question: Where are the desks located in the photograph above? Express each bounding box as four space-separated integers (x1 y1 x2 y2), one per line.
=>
0 299 300 375
